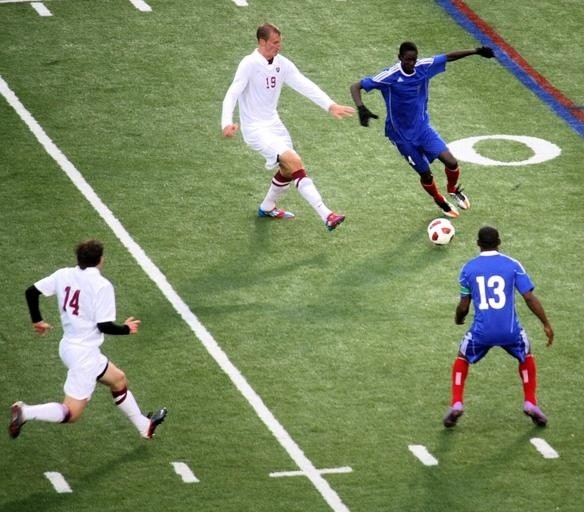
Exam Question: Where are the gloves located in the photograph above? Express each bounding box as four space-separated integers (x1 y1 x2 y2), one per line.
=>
357 103 379 128
477 45 497 58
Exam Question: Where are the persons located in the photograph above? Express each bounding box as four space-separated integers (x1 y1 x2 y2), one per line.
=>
441 224 555 427
220 23 356 232
349 41 496 218
9 238 168 440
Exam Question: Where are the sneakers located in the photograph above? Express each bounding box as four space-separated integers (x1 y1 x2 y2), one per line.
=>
435 193 459 219
259 208 296 220
447 183 472 213
443 402 463 427
325 214 344 232
8 401 27 440
145 407 168 439
521 401 548 428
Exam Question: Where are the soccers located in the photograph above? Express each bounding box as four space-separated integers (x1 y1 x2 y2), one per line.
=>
427 218 454 244
